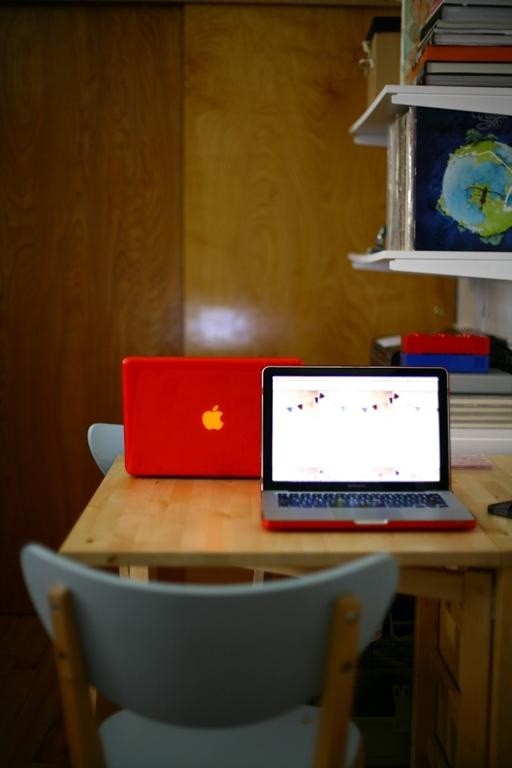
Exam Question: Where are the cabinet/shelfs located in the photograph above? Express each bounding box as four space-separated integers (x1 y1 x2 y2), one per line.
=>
348 83 512 280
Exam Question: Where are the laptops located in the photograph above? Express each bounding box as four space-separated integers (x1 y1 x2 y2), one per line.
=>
122 356 304 479
260 365 477 531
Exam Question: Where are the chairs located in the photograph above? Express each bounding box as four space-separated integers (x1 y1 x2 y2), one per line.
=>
87 423 125 476
20 543 400 768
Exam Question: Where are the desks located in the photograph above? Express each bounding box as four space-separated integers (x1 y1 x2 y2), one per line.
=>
55 452 500 768
450 454 512 768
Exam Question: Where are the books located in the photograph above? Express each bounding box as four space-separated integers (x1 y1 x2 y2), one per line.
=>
404 0 512 88
